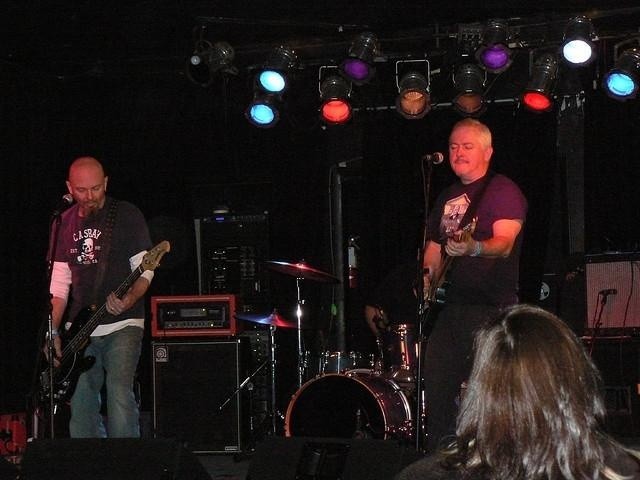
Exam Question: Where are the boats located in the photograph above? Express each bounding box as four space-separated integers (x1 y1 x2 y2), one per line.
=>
580 337 640 439
245 436 406 480
152 330 268 453
19 437 213 480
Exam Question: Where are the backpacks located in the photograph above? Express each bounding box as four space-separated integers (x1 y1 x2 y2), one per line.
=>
285 369 412 439
379 321 417 399
316 346 384 373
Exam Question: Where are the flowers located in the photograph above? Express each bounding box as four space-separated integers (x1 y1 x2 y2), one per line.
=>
422 152 444 165
600 289 618 295
51 194 73 216
333 153 377 169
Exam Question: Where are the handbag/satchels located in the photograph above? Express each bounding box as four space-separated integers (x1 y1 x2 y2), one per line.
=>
468 239 483 257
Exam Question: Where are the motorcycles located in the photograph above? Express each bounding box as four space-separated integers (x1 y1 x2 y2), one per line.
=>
250 361 287 455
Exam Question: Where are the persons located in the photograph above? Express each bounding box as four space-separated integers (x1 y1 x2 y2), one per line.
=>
355 250 411 357
394 301 640 479
43 155 153 440
409 114 528 452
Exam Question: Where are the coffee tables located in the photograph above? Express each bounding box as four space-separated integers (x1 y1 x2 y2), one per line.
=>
233 311 311 329
262 259 341 284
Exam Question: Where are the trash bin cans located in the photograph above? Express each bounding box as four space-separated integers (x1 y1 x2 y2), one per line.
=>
43 330 59 341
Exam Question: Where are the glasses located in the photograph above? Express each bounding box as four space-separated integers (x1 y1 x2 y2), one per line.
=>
478 19 512 70
451 41 482 116
603 37 640 101
561 17 594 64
343 32 377 81
247 93 283 130
315 66 353 123
525 44 555 109
395 61 431 119
258 43 295 94
190 40 233 88
